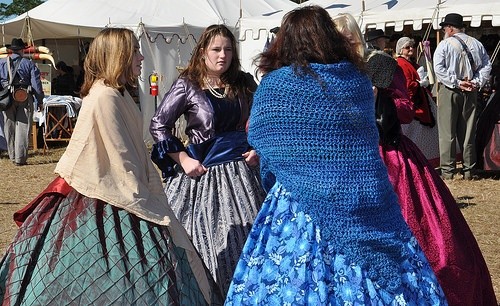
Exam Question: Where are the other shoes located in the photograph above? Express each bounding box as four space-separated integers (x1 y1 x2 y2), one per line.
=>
10 160 28 167
441 171 481 181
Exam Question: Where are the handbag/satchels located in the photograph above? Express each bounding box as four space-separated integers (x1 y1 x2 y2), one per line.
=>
12 87 30 104
0 85 11 110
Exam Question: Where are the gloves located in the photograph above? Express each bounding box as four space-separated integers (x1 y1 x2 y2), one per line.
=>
416 76 430 88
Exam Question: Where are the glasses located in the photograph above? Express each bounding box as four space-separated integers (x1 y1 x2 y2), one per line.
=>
402 45 414 50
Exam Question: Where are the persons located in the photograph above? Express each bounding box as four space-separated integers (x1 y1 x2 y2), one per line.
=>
328 12 497 306
224 5 449 306
148 25 268 302
391 37 462 168
51 61 78 97
0 27 224 306
475 59 500 173
433 13 493 181
365 29 422 63
0 38 46 166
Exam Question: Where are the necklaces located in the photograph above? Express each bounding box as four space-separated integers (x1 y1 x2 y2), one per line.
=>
201 73 232 99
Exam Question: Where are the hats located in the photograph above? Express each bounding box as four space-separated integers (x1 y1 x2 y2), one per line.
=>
439 13 472 28
365 29 390 42
5 38 28 50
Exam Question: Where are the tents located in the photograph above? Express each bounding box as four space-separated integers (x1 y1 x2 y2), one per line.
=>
238 0 500 166
0 0 299 153
